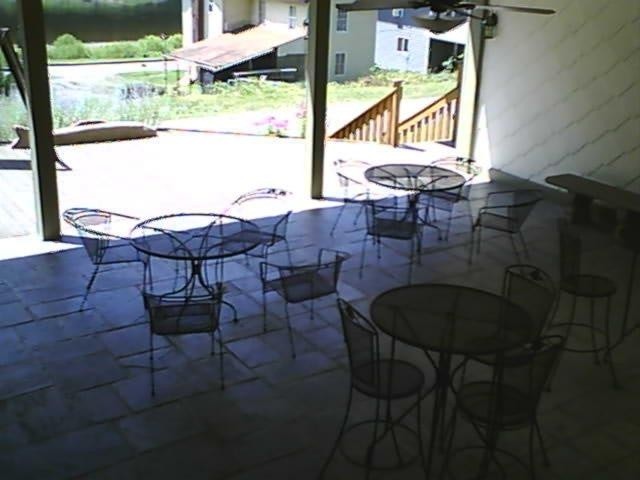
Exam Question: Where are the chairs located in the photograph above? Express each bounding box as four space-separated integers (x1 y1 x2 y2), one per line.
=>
63 207 153 312
143 281 226 397
259 248 352 360
220 187 295 280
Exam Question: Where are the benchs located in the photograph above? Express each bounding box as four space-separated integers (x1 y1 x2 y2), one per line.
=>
545 173 640 240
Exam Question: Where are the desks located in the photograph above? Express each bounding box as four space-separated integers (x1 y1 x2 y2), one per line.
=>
129 213 262 323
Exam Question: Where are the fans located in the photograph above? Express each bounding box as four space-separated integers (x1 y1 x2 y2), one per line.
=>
336 0 556 21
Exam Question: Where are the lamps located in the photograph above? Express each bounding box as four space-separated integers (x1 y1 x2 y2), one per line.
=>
411 5 467 34
480 13 498 38
